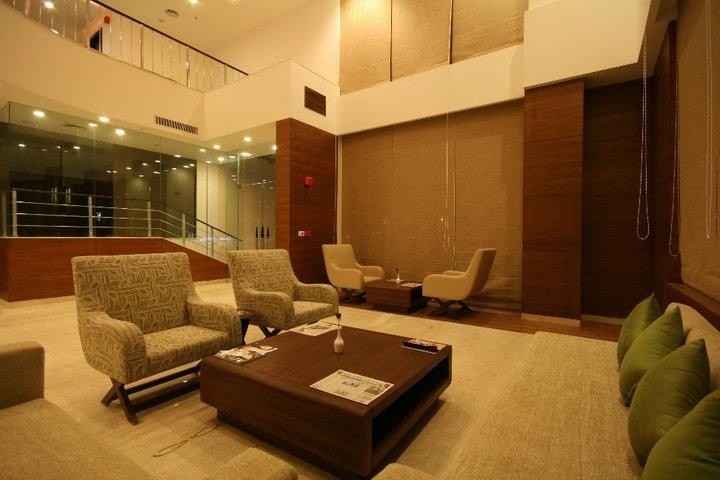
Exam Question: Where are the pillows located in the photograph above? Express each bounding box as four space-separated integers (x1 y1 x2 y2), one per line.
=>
615 305 685 406
614 292 663 369
626 337 711 468
640 387 720 480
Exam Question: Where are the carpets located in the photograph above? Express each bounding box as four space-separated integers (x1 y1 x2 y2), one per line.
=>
1 281 533 480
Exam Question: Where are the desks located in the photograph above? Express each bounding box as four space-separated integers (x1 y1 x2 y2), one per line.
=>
235 308 256 345
367 280 428 314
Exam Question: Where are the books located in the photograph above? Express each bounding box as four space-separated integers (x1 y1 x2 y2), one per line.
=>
401 338 447 356
213 342 279 366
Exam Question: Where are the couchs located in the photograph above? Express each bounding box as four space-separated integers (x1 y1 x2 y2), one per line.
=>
369 301 719 480
1 338 298 479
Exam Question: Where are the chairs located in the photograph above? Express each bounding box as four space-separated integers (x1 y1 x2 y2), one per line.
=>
225 247 342 338
322 243 386 297
70 250 244 425
422 248 498 322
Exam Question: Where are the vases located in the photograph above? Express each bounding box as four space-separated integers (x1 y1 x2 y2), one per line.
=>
334 331 345 354
396 274 401 285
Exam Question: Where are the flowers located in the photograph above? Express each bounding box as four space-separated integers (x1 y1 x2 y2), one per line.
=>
335 311 342 332
396 268 401 274
